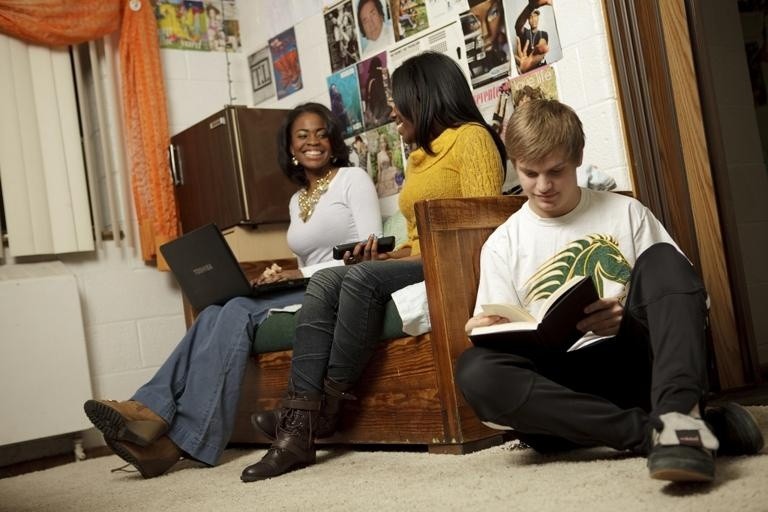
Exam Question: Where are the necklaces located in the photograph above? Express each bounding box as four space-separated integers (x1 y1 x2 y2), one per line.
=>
297 169 336 223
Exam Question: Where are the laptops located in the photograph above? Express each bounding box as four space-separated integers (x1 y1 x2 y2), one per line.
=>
159 223 310 312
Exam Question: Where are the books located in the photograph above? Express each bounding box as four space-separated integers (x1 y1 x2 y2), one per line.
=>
465 272 601 359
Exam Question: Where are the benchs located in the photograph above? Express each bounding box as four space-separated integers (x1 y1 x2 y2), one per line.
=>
177 191 638 454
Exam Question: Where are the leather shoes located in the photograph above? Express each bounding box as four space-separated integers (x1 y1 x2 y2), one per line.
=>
104 434 182 477
83 398 169 449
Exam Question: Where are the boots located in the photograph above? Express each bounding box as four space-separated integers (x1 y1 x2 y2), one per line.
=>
249 402 339 440
239 409 318 482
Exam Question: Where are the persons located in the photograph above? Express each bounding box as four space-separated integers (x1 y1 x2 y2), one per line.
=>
82 100 383 480
363 56 387 119
325 5 356 63
512 0 553 72
206 2 225 40
487 82 512 136
454 95 764 486
350 135 368 172
398 0 417 29
464 0 502 52
356 0 395 58
374 135 399 198
328 83 350 134
237 47 509 486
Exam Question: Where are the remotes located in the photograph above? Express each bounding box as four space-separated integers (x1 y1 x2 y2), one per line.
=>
333 236 395 260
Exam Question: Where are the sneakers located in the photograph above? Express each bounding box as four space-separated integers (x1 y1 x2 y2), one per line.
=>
704 401 765 455
647 408 721 482
